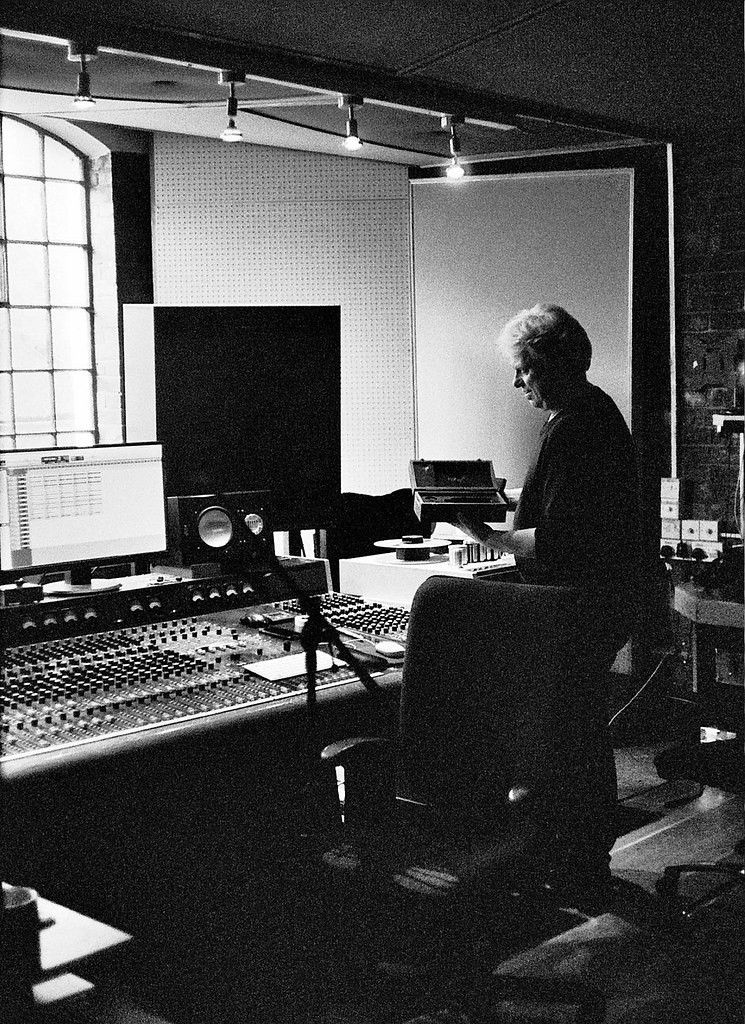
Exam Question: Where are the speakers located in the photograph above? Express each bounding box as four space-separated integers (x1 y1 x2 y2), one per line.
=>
167 489 278 578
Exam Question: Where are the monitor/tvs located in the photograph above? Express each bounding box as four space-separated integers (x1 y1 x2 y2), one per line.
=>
0 440 170 595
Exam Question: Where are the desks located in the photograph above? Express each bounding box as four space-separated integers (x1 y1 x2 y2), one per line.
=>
0 882 135 1024
340 552 517 612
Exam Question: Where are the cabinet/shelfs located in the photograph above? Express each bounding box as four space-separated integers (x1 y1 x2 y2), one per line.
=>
657 579 745 783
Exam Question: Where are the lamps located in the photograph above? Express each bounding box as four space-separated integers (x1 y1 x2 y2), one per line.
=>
338 96 364 152
442 117 467 181
67 43 97 112
218 71 245 143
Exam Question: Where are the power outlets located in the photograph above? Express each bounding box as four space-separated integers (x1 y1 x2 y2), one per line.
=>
660 539 723 564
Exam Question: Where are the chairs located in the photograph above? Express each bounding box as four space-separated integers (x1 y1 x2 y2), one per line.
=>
294 574 620 1024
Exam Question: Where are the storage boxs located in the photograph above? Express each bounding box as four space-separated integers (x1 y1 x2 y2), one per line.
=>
410 459 507 523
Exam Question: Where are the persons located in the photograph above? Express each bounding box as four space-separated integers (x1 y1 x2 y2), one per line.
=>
451 306 640 905
